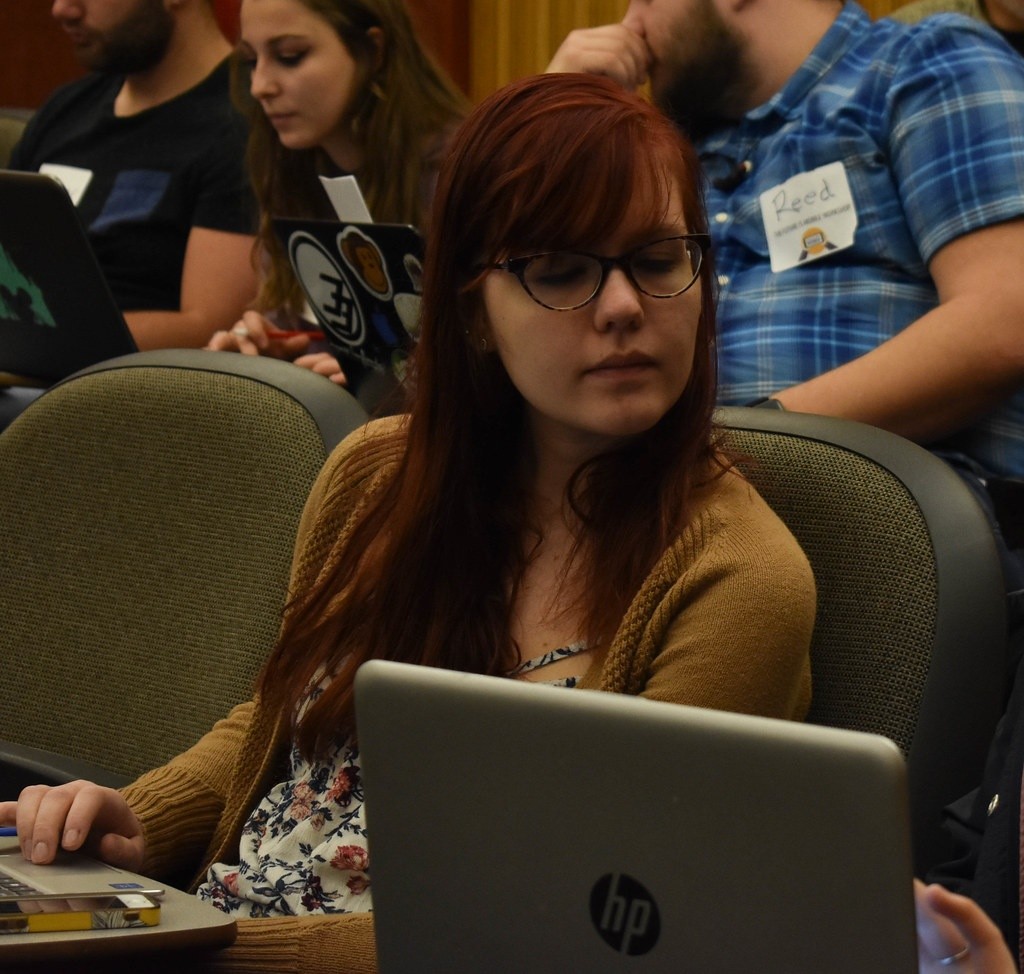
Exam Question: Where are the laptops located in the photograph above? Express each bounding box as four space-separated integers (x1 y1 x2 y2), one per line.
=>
0 848 238 973
272 214 428 397
351 659 921 974
0 169 140 383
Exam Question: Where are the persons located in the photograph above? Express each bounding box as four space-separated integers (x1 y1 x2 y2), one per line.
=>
0 2 276 389
0 68 823 974
914 876 1018 974
546 2 1022 524
200 0 492 383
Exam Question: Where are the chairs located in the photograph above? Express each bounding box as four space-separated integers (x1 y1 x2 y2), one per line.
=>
0 350 371 806
714 405 1002 809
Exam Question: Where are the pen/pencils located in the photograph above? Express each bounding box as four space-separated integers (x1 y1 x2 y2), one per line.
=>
234 328 327 341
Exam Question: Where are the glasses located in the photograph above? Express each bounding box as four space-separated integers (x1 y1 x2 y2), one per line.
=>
478 233 712 314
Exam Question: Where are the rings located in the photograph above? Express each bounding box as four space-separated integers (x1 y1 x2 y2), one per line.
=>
939 944 971 967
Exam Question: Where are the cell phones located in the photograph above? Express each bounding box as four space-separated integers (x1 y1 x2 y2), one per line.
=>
0 888 162 935
